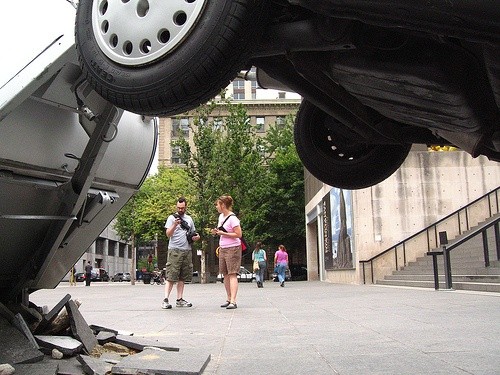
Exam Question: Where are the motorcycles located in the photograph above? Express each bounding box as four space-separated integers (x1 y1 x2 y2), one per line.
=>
150 270 165 285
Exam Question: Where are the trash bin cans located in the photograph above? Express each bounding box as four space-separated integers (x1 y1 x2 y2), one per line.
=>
143 274 152 284
192 271 200 284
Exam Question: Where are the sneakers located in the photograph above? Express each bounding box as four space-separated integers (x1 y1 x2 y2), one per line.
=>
176 298 192 307
161 300 172 309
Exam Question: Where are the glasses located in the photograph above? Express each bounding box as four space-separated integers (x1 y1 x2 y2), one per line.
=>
177 208 184 210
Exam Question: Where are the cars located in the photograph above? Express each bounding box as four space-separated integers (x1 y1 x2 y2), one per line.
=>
217 265 256 283
75 267 110 282
111 272 130 282
272 264 307 282
192 270 200 283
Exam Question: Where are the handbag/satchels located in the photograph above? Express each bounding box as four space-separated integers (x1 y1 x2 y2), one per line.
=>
242 241 247 251
274 265 280 274
254 261 260 272
187 230 197 244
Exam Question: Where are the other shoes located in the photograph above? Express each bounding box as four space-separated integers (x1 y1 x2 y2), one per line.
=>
221 300 230 307
280 280 285 287
257 282 263 288
226 303 237 309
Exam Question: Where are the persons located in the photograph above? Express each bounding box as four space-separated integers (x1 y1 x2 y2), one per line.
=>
210 196 242 309
161 196 200 309
252 242 268 288
274 245 288 287
85 261 92 286
69 266 76 286
